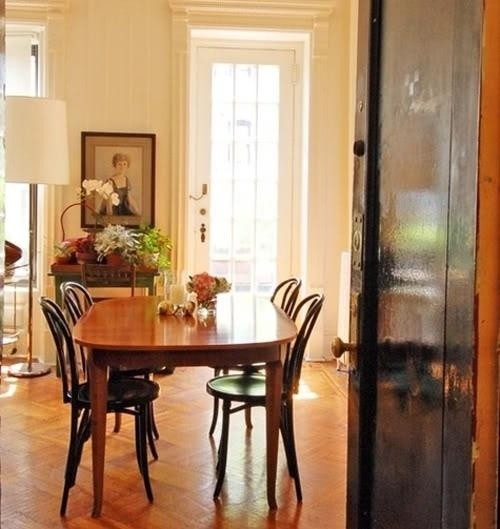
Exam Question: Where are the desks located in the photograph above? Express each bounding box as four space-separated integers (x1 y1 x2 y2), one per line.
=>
46 261 161 379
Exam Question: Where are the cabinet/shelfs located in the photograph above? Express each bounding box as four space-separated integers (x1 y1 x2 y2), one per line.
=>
334 250 350 372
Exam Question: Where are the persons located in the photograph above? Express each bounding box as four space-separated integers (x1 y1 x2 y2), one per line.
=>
98 152 138 216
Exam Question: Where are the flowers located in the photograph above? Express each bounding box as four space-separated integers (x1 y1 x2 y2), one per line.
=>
51 176 122 242
74 233 97 254
94 224 142 262
185 271 232 307
52 241 74 259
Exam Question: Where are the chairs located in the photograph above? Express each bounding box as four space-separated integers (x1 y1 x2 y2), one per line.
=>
37 294 160 517
80 259 136 316
207 275 303 435
57 281 159 462
206 291 326 504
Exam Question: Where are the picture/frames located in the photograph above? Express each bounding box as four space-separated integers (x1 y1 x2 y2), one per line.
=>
80 130 156 229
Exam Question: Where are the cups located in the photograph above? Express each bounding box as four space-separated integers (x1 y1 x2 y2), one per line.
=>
163 269 192 304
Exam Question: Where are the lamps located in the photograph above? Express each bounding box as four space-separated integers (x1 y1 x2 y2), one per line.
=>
4 95 71 378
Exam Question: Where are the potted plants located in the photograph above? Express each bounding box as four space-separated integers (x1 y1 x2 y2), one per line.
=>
131 222 173 270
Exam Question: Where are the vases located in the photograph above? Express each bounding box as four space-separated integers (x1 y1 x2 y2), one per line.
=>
56 255 71 265
196 295 217 318
75 252 97 265
106 251 124 265
